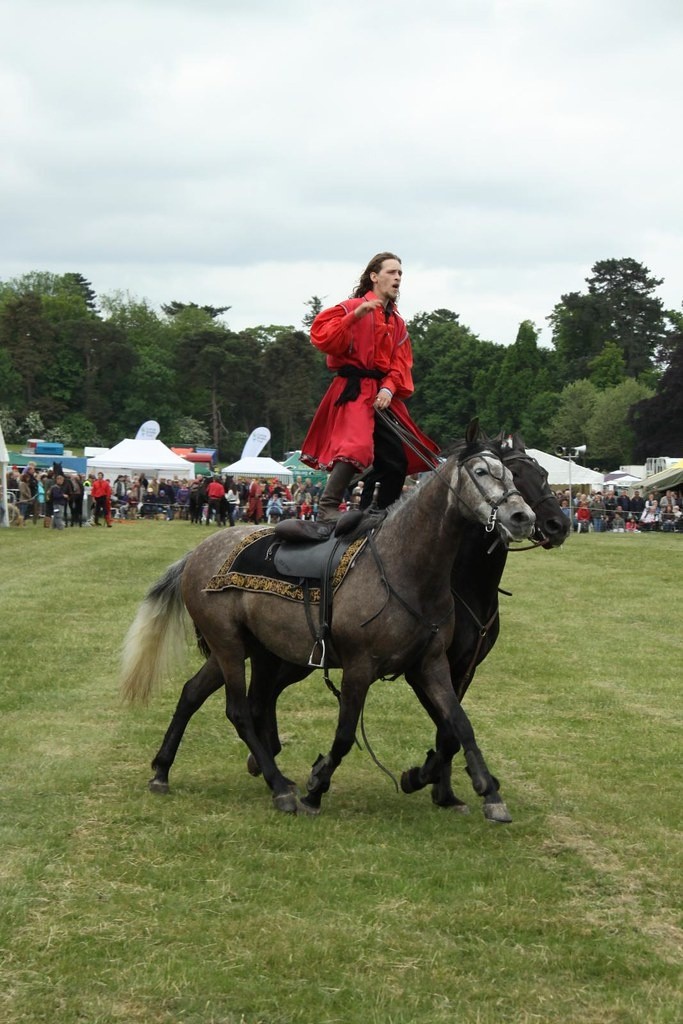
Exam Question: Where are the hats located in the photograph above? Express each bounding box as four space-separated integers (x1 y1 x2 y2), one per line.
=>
160 490 165 494
147 488 153 493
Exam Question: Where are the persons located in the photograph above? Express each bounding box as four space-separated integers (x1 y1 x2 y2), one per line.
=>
549 488 683 533
299 251 442 525
5 461 365 530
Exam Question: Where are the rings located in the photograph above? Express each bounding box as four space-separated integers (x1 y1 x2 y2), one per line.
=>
377 399 380 402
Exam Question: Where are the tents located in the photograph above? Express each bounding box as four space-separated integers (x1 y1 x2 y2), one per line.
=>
0 438 683 529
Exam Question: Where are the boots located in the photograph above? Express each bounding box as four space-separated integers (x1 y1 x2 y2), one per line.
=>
45 516 51 527
316 462 357 524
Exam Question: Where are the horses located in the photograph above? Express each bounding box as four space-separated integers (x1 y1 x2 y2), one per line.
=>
119 417 571 822
